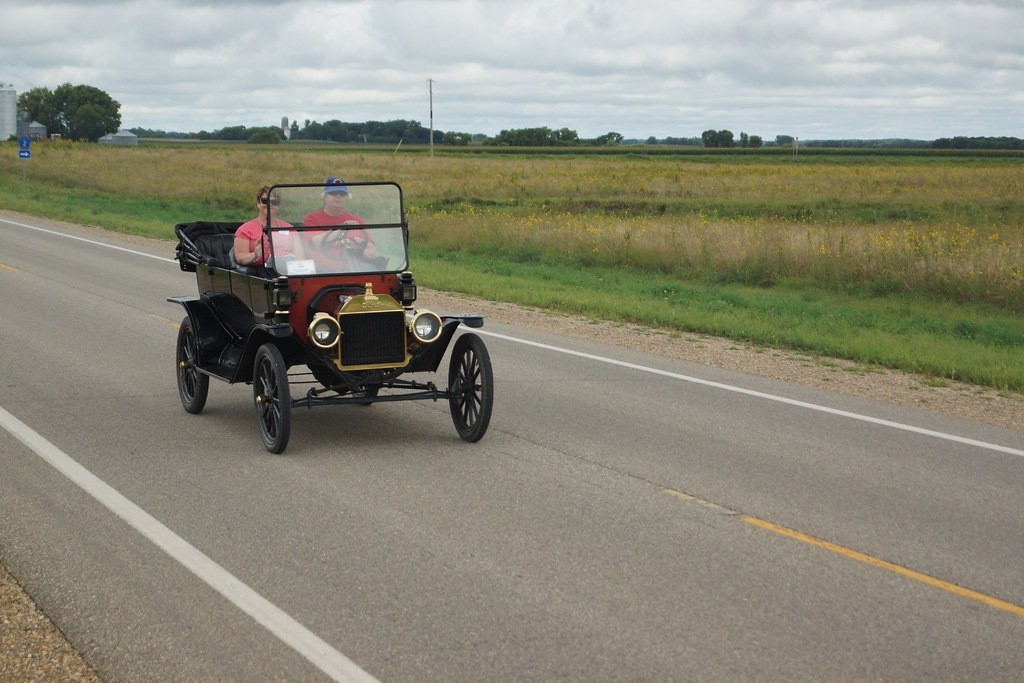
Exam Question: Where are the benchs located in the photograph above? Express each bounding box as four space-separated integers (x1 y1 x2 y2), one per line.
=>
198 225 301 267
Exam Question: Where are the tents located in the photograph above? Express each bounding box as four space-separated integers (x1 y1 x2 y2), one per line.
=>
98 131 137 145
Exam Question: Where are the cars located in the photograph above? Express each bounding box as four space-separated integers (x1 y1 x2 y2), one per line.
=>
165 181 494 455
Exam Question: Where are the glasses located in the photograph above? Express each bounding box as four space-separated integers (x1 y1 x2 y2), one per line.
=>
260 198 279 206
329 190 347 196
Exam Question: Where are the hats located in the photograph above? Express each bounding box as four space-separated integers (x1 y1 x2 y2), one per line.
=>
324 177 348 193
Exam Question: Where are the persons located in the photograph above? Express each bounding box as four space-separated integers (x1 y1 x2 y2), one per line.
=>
234 186 304 269
302 177 376 274
